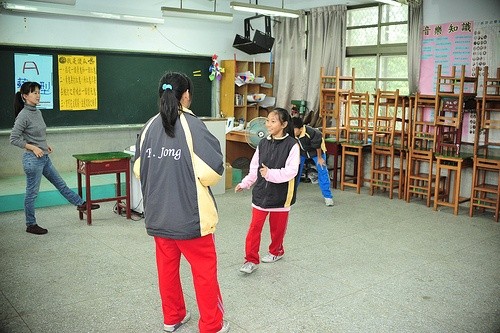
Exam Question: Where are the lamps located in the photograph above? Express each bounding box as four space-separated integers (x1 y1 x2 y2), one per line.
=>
230 0 300 19
160 0 234 23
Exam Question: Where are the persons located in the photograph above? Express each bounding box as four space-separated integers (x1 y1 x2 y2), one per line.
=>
9 82 100 234
133 72 229 333
234 109 333 274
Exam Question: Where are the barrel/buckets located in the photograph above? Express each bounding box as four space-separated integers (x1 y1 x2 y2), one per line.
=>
232 169 243 184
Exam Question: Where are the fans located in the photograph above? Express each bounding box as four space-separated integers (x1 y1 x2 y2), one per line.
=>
244 116 272 151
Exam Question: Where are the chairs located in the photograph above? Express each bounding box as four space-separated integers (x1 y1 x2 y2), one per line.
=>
312 64 500 223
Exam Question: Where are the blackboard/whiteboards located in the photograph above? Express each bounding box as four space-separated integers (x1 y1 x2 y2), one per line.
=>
0 42 215 134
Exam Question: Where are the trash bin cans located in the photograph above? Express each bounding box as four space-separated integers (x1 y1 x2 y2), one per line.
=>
225 161 232 190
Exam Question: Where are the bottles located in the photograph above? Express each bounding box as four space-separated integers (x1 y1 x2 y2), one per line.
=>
225 162 232 189
221 110 223 118
235 92 242 105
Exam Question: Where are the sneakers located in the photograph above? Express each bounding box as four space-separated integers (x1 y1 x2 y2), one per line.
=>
240 261 259 273
325 198 334 206
262 253 284 263
218 320 230 333
163 311 190 332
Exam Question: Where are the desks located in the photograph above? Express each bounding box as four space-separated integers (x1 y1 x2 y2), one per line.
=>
72 151 133 226
226 131 256 169
324 137 358 190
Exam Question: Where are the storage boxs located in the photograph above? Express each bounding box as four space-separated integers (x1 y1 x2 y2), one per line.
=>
232 167 244 183
227 163 233 189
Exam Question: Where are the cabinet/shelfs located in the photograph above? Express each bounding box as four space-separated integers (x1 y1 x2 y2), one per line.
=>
220 58 274 122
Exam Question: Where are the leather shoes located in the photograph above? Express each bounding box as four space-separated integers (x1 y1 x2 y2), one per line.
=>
77 201 100 210
26 224 48 235
